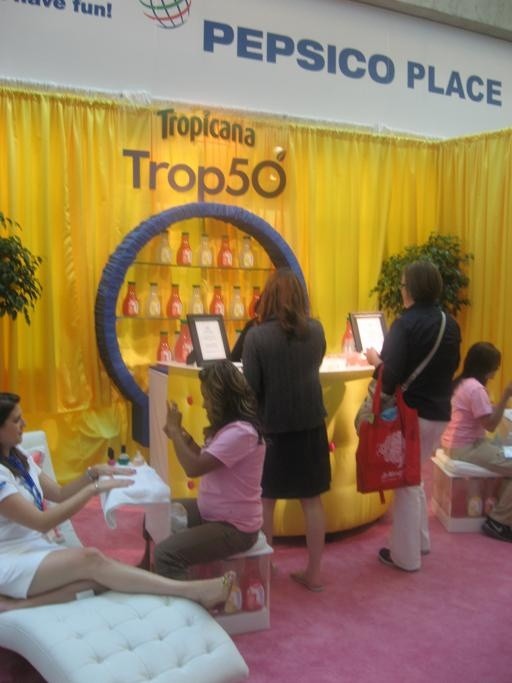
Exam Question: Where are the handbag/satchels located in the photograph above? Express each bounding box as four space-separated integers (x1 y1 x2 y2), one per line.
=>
354 313 446 437
355 362 422 494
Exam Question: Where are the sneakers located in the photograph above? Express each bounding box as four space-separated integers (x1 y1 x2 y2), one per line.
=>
204 571 235 617
378 547 395 564
482 516 512 541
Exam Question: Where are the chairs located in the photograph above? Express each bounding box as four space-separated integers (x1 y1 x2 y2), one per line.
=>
1 428 248 683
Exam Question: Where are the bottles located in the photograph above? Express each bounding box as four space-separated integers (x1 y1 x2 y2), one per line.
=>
174 319 193 363
248 286 260 318
122 280 139 317
156 331 172 361
197 233 213 267
188 284 203 315
118 446 129 465
228 285 244 319
107 447 115 466
218 235 232 266
176 232 192 265
210 285 225 316
156 230 172 263
239 236 254 268
144 282 161 317
166 284 183 317
341 317 355 354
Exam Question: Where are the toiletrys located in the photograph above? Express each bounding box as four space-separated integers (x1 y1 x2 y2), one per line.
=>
106 446 116 467
117 445 130 465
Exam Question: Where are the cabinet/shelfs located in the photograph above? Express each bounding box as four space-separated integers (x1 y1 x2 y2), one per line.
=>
148 358 392 538
118 259 275 322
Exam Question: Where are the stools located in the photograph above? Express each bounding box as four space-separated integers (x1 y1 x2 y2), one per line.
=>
428 457 503 532
185 539 270 633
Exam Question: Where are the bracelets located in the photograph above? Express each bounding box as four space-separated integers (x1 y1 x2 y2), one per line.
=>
84 465 98 480
94 481 100 491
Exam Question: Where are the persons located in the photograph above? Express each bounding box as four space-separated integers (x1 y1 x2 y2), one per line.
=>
139 359 267 581
441 341 512 543
243 268 331 591
0 391 236 614
362 260 461 571
230 301 259 361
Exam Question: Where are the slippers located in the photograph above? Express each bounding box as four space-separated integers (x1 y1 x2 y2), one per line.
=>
291 567 324 592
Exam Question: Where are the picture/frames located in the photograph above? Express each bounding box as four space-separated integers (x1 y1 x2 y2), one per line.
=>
186 313 231 366
349 312 387 356
